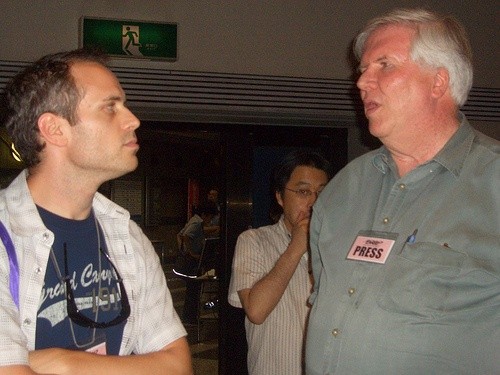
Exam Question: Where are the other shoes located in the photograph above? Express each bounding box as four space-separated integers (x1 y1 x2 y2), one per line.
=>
182 315 203 324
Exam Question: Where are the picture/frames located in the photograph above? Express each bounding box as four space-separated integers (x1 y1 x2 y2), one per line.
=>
145 175 187 227
110 176 144 224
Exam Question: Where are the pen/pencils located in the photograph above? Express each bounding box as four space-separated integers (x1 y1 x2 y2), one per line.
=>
400 229 418 254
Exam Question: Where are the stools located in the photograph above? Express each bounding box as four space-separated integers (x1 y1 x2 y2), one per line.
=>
171 237 225 343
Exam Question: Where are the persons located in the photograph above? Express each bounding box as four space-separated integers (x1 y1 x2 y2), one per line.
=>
301 7 500 375
204 188 220 235
176 199 218 326
228 149 330 375
0 45 192 375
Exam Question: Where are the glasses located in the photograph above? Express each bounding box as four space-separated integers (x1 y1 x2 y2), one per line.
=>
64 241 131 328
283 186 325 197
